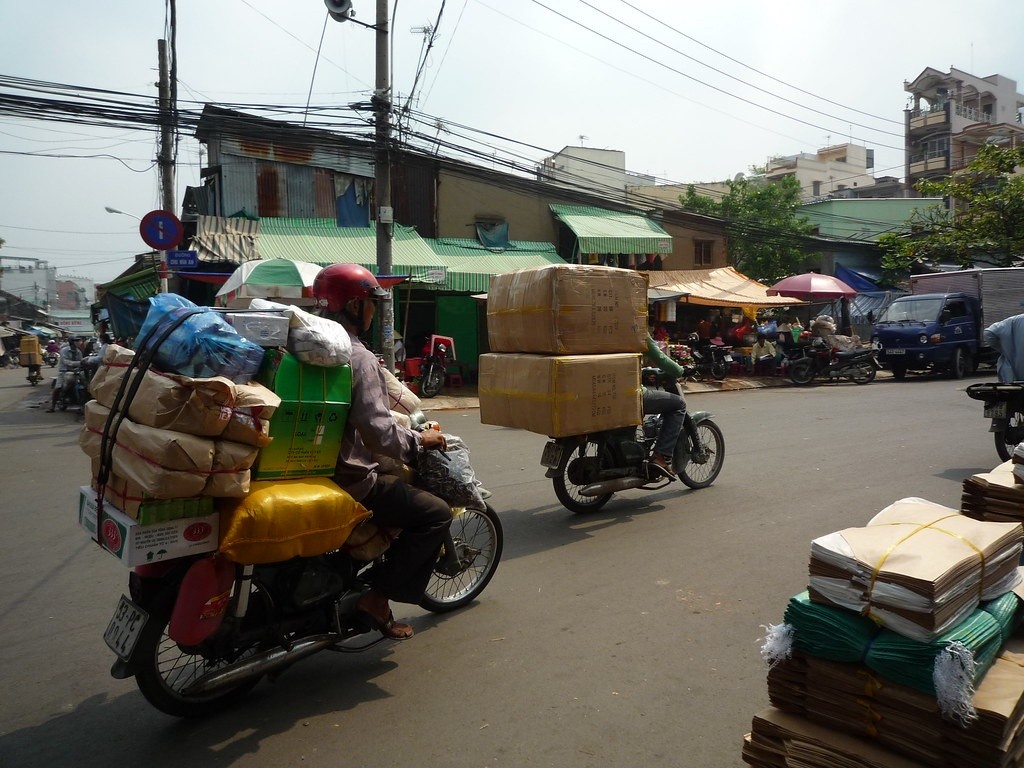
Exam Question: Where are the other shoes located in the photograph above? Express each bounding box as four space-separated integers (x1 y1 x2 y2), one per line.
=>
45 408 55 412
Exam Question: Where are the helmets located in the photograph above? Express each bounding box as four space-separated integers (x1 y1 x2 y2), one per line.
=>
48 340 55 343
312 262 388 312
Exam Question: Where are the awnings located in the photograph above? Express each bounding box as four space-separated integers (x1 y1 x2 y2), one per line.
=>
0 321 74 338
547 203 673 263
89 302 109 326
817 262 910 325
646 288 691 304
639 265 811 308
188 213 570 293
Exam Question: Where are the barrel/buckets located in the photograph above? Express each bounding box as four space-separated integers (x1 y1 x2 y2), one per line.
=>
792 329 804 344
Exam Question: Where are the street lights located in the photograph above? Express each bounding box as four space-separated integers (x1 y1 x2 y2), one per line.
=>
103 205 180 295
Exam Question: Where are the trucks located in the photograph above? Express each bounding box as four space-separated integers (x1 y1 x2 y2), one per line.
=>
869 267 1024 381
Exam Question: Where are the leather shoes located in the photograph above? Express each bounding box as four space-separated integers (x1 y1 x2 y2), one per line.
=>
356 588 414 641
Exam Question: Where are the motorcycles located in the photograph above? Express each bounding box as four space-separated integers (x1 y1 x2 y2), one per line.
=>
538 365 726 514
964 380 1023 463
416 336 453 399
688 330 734 381
47 351 60 367
53 365 89 411
104 404 504 724
25 355 45 386
790 338 882 386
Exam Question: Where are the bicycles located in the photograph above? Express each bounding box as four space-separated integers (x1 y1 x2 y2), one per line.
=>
4 350 20 369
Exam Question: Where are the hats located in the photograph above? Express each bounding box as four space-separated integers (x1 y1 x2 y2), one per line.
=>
68 336 81 341
710 337 725 345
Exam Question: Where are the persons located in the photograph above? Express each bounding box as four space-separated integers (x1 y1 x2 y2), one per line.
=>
983 314 1024 384
640 330 694 481
646 374 656 386
313 263 453 641
748 334 777 377
45 334 112 414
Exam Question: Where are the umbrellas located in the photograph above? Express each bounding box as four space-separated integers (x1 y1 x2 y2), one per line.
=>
766 271 857 319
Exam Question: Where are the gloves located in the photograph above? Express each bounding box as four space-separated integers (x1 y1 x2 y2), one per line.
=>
683 365 697 377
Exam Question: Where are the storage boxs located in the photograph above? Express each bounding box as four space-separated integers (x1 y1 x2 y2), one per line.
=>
20 352 39 365
90 459 218 524
478 353 643 438
251 348 351 482
79 485 218 568
21 335 39 352
227 312 289 347
486 263 649 355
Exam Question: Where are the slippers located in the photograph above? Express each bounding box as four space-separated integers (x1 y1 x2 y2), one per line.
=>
649 461 678 481
